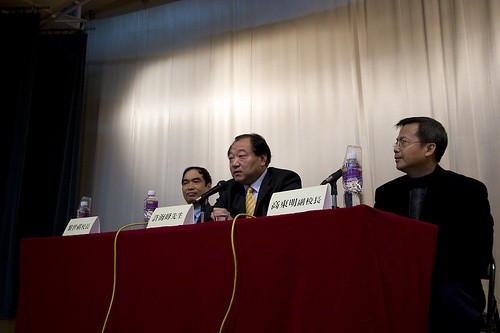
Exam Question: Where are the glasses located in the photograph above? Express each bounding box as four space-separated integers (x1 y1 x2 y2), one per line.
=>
394 138 432 147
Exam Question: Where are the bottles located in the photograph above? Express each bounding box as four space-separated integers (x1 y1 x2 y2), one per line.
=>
342 154 364 207
143 190 158 228
76 201 90 218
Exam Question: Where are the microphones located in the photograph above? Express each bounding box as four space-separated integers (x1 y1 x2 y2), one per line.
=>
192 180 228 204
324 167 343 183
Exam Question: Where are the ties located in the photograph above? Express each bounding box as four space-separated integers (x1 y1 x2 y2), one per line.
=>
245 186 256 217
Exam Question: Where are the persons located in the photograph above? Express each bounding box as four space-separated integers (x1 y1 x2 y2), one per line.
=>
211 133 301 221
374 117 493 333
182 166 212 222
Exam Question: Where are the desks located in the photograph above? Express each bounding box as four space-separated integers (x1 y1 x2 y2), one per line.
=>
18 204 437 333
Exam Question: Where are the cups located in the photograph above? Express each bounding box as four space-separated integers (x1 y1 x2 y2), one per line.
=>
341 145 362 171
77 196 92 212
